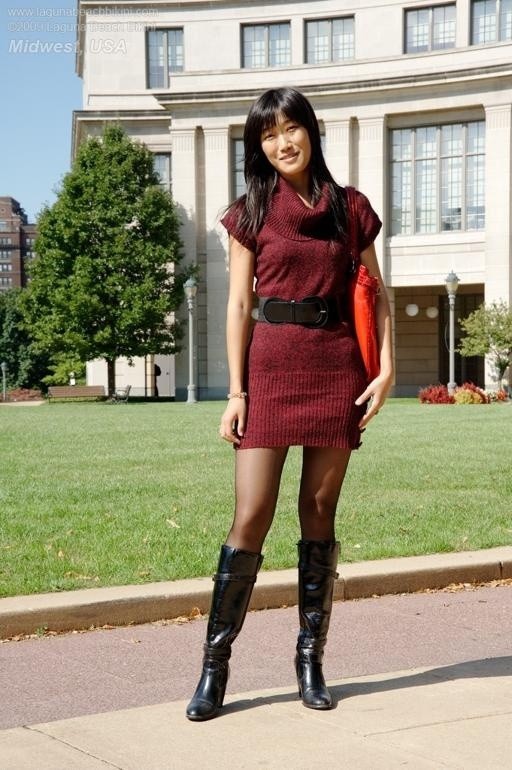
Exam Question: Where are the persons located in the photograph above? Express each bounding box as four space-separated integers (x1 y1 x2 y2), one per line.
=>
182 88 397 720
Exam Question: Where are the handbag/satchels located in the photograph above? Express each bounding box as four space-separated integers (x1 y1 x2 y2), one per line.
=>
344 183 380 385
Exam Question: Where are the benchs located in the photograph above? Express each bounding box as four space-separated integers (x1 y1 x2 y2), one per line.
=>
48 386 105 404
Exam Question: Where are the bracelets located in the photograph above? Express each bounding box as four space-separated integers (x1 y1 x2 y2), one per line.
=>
226 391 248 399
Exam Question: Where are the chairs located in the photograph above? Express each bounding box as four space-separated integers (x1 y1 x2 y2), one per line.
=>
113 385 131 405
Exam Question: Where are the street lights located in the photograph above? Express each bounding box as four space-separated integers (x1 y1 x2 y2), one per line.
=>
183 278 199 403
445 271 460 394
1 361 7 400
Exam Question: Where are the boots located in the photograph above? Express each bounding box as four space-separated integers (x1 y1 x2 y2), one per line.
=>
182 547 264 721
291 539 342 708
